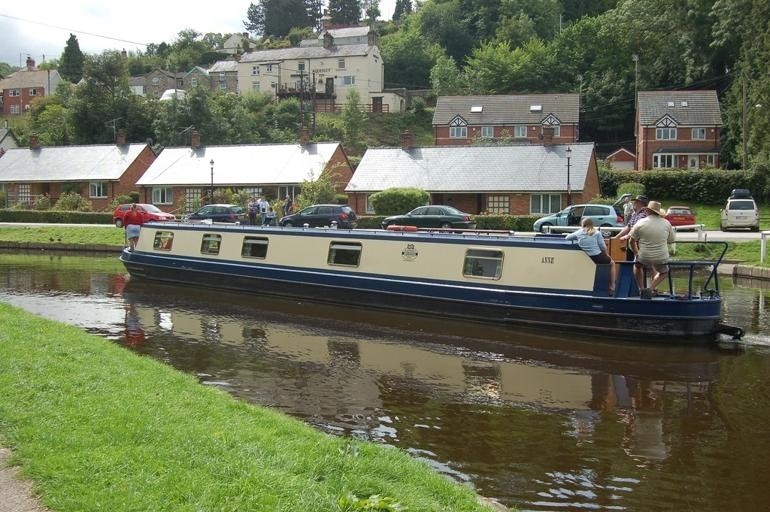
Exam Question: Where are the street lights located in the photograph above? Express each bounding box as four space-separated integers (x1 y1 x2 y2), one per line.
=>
210 159 214 204
565 147 572 206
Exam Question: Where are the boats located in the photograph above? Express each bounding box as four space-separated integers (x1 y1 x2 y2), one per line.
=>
120 219 745 341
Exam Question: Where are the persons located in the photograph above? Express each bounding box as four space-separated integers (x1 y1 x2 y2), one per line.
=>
611 195 659 294
124 301 146 353
567 372 671 481
630 199 676 294
123 202 145 252
564 215 616 296
621 196 634 227
247 193 295 228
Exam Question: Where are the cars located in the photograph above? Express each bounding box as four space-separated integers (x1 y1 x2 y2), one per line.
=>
720 189 759 231
533 194 632 237
112 203 242 228
279 204 357 229
381 205 476 233
664 206 695 232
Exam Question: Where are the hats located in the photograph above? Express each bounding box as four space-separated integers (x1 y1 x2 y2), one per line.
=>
631 194 649 203
640 200 665 216
